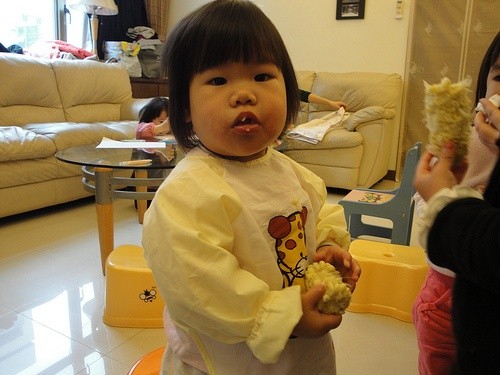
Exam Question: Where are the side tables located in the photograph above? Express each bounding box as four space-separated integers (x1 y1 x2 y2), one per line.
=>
128 76 171 99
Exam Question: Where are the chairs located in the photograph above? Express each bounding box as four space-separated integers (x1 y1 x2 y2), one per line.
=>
338 141 422 247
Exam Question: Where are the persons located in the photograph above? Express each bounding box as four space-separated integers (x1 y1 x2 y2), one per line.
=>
128 97 171 210
299 89 347 111
415 98 500 375
411 31 500 375
143 0 361 375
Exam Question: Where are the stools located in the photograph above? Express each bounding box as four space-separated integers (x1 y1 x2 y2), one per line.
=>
103 245 166 329
345 240 430 324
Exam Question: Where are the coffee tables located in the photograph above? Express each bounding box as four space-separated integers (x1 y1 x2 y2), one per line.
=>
55 138 288 276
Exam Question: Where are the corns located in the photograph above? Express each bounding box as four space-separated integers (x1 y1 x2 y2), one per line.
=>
303 261 353 314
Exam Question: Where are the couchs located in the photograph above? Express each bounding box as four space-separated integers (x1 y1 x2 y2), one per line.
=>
275 71 403 190
0 51 170 219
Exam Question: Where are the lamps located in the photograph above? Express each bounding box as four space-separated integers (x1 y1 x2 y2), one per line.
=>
68 0 119 61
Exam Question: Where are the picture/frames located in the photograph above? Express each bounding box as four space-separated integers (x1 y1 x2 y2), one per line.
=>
336 0 365 20
